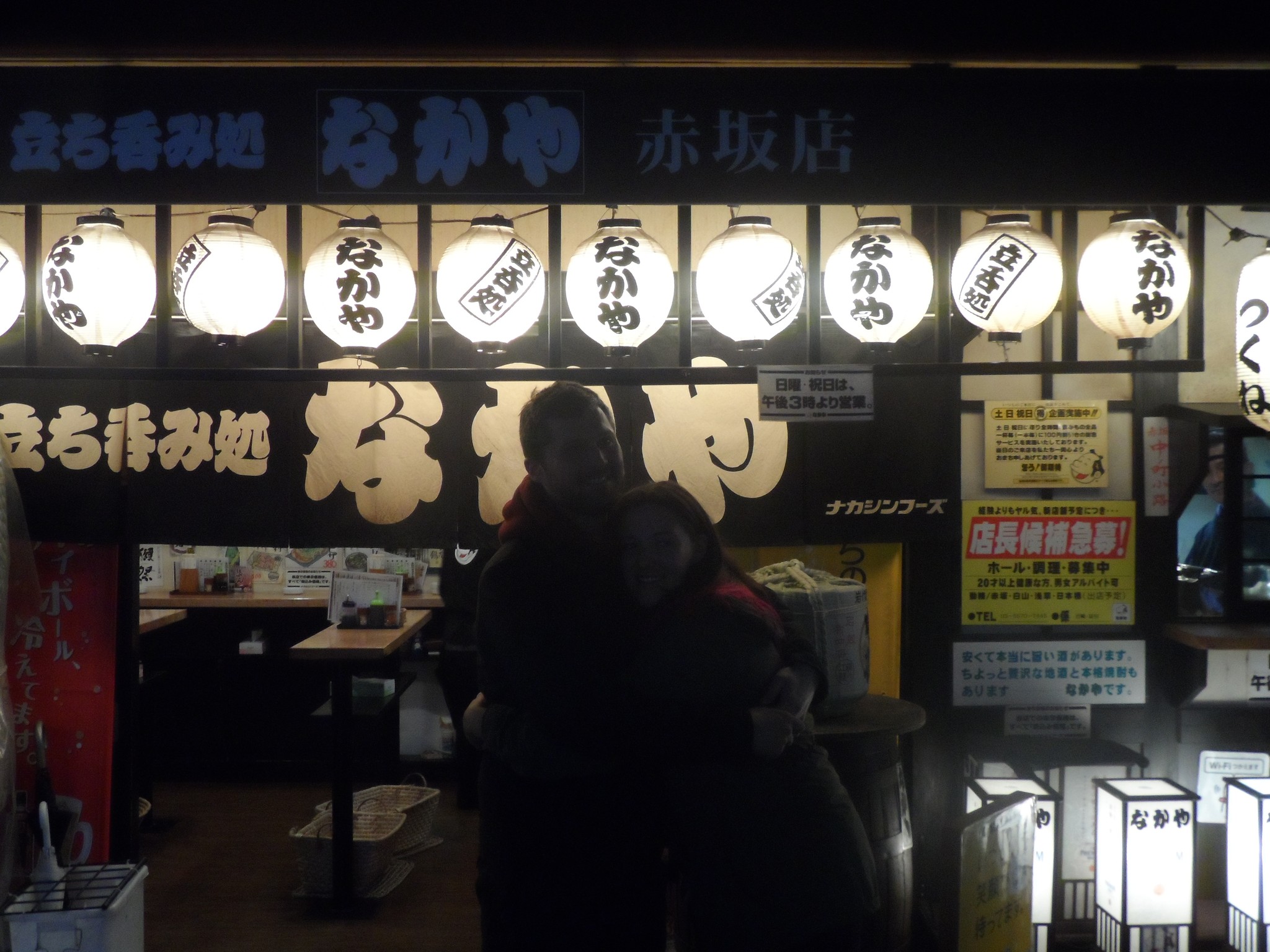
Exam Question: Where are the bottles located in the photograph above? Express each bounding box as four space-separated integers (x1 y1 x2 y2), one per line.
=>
211 565 229 595
356 606 370 629
204 577 214 595
368 590 384 629
341 595 358 629
382 604 397 629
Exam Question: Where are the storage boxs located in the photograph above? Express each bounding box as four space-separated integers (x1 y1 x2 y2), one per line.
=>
5 863 150 952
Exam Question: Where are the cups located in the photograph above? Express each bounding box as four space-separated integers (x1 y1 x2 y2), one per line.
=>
369 569 387 574
179 569 199 594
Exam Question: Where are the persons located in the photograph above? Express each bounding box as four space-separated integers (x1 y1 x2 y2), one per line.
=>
1202 430 1270 570
474 383 883 952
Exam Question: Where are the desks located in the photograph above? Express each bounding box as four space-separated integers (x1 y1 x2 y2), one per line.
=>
140 585 446 607
138 609 187 835
291 607 434 920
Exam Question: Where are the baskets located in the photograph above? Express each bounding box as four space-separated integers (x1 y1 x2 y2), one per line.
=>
313 772 441 856
288 798 406 896
